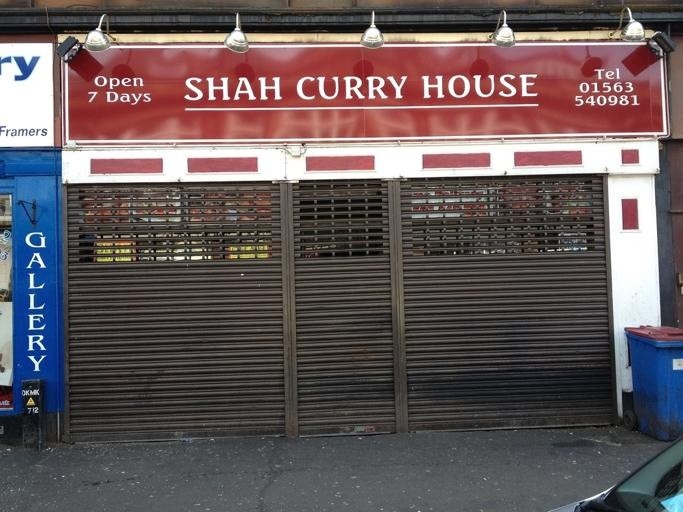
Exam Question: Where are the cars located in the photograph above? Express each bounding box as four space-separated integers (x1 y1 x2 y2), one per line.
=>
546 431 683 511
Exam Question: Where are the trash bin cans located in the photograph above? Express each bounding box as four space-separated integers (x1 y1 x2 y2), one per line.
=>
623 326 683 441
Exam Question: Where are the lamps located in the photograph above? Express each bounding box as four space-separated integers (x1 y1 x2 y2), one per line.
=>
224 13 249 53
610 7 645 42
55 36 83 63
85 13 116 53
360 10 384 49
645 31 677 59
488 9 515 48
